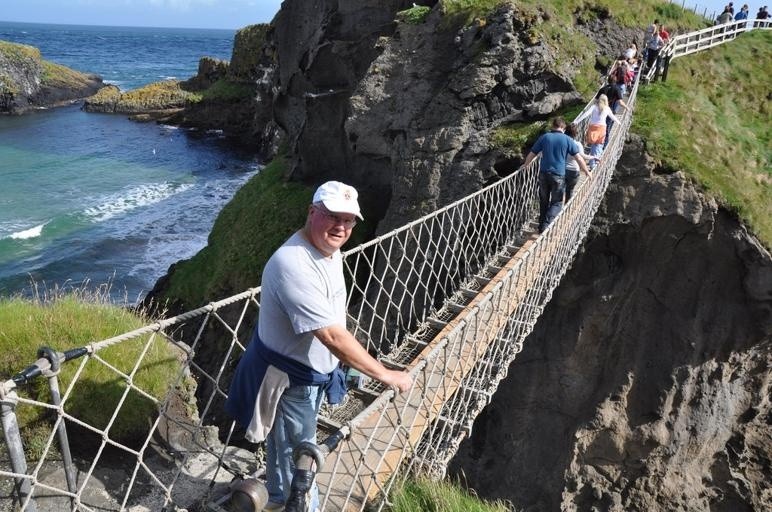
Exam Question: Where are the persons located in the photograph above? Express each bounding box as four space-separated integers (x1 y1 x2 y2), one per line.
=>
221 179 414 511
520 117 593 237
713 0 772 44
587 18 671 147
563 123 591 206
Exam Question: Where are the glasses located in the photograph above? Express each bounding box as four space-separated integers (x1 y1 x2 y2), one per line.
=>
312 205 356 228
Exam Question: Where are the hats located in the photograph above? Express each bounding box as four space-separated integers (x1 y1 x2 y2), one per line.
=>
311 180 367 222
